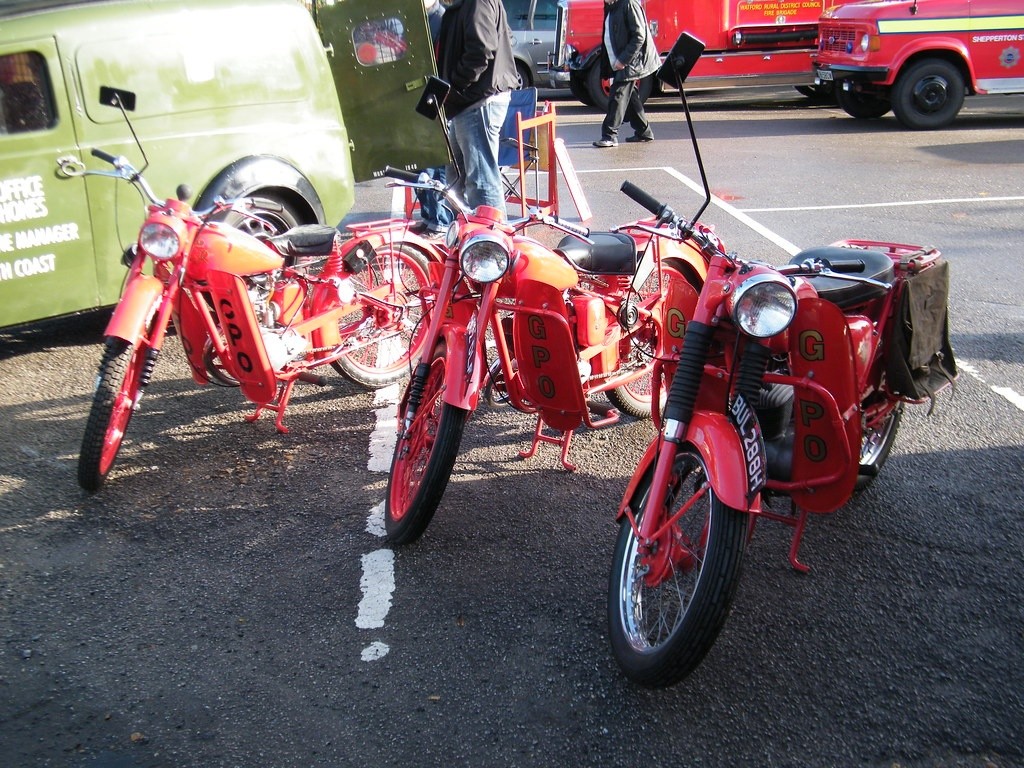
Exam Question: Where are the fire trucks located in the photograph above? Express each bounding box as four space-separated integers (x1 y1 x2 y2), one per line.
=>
796 1 1024 132
542 0 864 114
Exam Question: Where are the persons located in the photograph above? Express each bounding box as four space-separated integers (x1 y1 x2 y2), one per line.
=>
589 0 662 147
424 0 519 221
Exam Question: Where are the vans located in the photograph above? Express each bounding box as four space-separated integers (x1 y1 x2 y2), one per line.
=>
1 0 453 336
501 0 572 92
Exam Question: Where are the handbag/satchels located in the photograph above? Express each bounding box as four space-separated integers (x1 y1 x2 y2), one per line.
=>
889 261 955 416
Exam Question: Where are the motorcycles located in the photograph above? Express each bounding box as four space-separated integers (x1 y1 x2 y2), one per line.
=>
607 32 963 693
385 73 727 547
77 85 449 493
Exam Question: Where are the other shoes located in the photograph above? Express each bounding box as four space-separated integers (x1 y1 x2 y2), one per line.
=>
409 221 427 235
419 229 446 241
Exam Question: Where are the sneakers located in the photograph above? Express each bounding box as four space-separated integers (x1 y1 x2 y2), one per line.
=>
625 134 653 143
593 136 619 147
